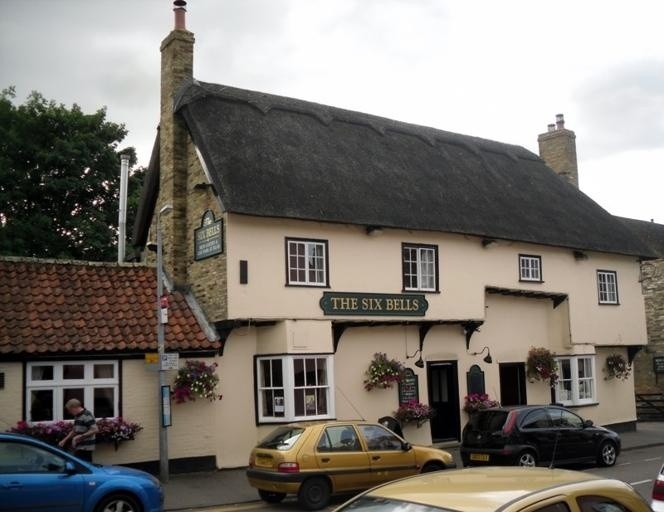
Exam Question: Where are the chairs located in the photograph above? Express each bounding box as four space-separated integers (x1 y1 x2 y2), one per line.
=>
339 429 355 451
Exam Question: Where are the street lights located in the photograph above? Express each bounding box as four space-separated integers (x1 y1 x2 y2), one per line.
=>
155 202 173 486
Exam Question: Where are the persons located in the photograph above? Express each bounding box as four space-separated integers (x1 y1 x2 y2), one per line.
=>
58 398 98 461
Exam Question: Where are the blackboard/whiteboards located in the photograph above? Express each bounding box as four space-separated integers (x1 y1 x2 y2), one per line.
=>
467 365 485 394
398 368 419 404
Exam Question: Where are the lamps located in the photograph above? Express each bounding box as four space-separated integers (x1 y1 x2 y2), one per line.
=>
544 295 568 309
481 238 496 250
573 250 587 261
367 225 383 238
473 347 492 363
407 349 424 368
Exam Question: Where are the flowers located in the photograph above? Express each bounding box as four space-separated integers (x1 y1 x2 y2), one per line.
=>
393 398 436 428
173 358 222 403
363 352 405 391
527 346 558 388
463 392 501 416
5 417 143 454
601 351 631 384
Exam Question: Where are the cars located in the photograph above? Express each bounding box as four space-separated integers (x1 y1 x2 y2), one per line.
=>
0 428 167 512
650 464 664 512
329 461 655 511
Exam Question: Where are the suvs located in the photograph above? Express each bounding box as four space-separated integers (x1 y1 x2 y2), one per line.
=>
244 416 457 511
456 403 625 471
